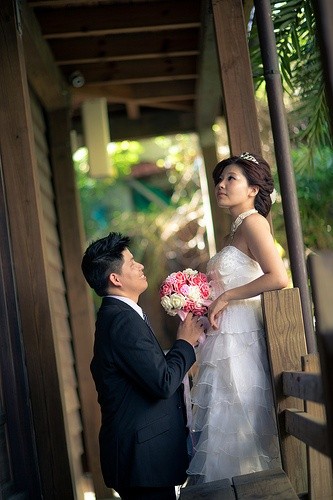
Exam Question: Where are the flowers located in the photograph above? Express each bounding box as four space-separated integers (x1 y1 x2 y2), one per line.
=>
161 268 214 344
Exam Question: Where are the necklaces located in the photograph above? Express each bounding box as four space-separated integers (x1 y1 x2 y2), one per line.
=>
225 207 261 248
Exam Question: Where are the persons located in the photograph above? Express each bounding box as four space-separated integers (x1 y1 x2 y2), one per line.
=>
80 232 207 500
176 153 292 486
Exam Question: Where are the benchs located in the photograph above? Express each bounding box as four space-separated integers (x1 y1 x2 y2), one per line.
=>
179 467 301 500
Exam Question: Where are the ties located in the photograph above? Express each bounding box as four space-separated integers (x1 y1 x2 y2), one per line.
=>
143 312 152 329
